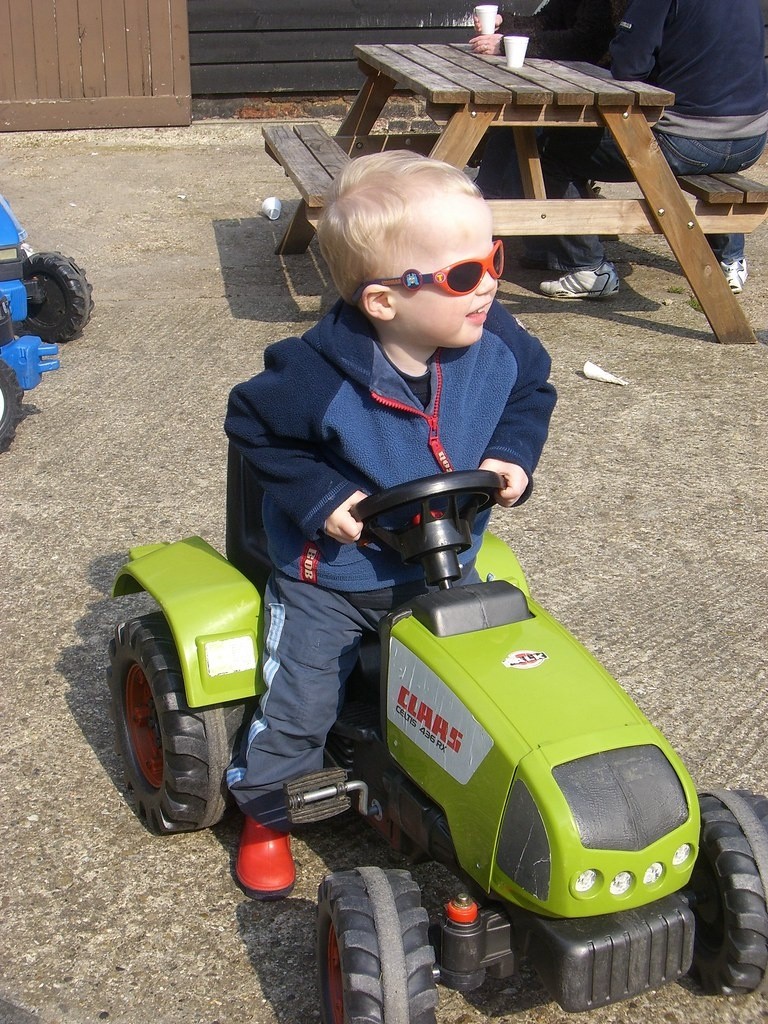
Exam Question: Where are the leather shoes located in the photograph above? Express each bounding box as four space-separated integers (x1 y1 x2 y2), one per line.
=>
236 813 297 900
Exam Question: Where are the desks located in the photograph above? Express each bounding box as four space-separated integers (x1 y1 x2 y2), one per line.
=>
276 44 754 344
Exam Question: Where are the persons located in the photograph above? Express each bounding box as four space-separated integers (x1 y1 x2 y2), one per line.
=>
540 0 768 302
464 1 634 269
221 147 557 902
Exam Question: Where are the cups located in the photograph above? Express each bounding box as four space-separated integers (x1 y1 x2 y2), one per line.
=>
503 36 529 68
474 4 498 35
261 197 282 220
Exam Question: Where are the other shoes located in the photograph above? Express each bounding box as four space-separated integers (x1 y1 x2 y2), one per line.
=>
521 252 548 269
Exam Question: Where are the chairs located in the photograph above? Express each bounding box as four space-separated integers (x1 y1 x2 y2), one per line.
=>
226 441 273 595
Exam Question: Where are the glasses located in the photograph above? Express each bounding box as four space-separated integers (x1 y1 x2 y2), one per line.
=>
351 240 503 305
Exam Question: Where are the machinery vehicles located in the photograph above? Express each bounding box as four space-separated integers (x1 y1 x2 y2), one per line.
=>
103 470 768 1024
0 196 94 463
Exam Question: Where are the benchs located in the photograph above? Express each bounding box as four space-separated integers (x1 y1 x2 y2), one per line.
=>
262 122 355 208
680 172 768 204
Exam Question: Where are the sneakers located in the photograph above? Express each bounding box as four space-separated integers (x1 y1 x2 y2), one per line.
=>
720 257 747 293
541 263 620 298
